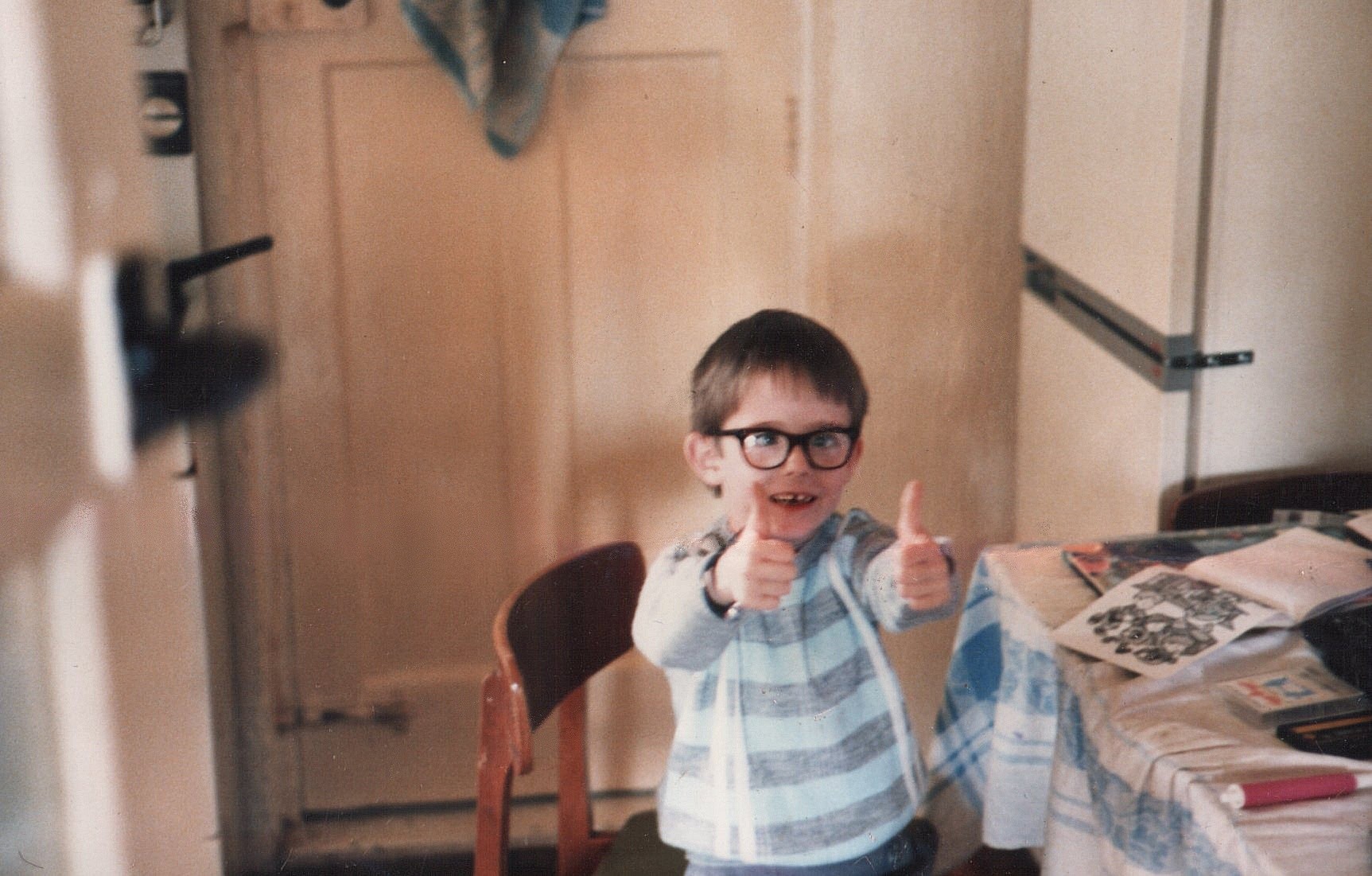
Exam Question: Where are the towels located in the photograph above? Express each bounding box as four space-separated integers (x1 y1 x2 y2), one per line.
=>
395 0 608 157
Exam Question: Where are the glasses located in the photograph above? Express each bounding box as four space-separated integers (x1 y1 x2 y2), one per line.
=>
709 426 860 471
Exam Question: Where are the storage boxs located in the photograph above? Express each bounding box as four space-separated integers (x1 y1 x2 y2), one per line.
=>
1219 666 1371 726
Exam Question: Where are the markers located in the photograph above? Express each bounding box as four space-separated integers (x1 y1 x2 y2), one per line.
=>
1219 771 1372 809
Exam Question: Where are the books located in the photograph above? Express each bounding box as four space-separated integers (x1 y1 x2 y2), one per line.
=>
1049 513 1372 730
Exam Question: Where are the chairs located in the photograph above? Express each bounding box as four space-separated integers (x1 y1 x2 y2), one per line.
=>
1159 458 1371 533
471 541 690 876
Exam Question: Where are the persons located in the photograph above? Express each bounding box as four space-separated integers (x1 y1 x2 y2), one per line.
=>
632 309 962 876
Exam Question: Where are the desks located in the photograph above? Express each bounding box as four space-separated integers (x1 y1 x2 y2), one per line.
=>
924 507 1371 874
